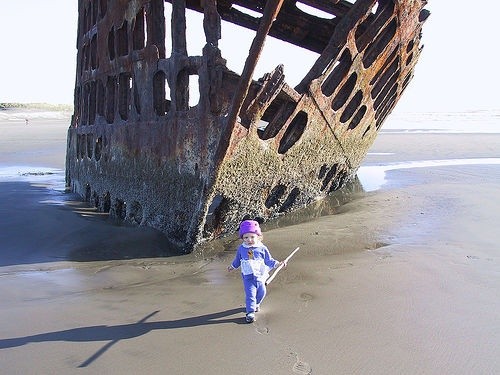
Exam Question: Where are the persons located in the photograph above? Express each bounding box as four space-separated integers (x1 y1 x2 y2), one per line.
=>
227 220 287 322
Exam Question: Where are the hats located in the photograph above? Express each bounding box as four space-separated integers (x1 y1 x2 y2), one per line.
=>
239 220 261 238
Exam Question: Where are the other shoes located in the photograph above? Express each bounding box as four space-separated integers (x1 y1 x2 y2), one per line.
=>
246 312 256 322
256 305 261 312
